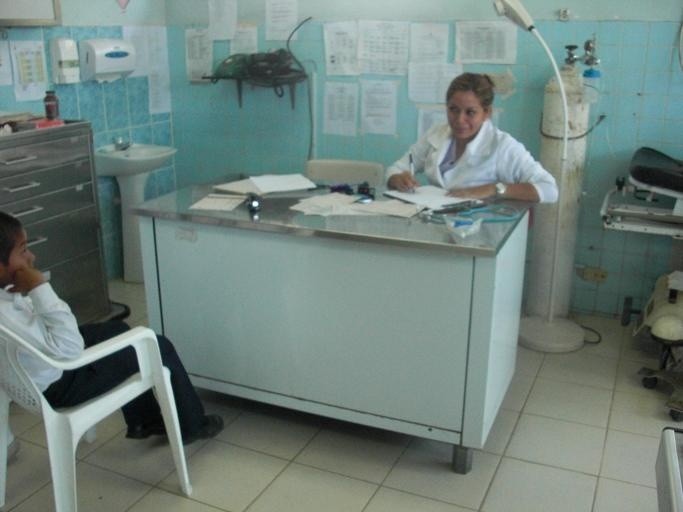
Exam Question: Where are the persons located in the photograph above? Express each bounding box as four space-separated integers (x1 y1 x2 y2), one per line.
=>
0 212 224 446
385 74 559 204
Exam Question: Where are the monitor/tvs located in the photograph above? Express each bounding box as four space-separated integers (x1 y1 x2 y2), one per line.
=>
213 179 309 198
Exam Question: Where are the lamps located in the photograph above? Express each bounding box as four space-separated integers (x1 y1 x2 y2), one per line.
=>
0 311 192 512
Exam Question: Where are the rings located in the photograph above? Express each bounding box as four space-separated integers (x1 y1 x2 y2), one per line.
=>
409 153 415 193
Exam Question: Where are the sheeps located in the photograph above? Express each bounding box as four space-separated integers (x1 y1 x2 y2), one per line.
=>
113 135 133 151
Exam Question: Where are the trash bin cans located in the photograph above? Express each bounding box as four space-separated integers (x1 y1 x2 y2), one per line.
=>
492 182 506 200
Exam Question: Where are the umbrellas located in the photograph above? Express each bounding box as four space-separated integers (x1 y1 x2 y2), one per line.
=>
182 415 223 444
126 425 149 440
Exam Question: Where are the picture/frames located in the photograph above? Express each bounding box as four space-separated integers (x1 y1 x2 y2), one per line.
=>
131 176 534 473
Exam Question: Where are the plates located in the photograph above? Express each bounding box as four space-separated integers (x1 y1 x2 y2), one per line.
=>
0 115 109 318
